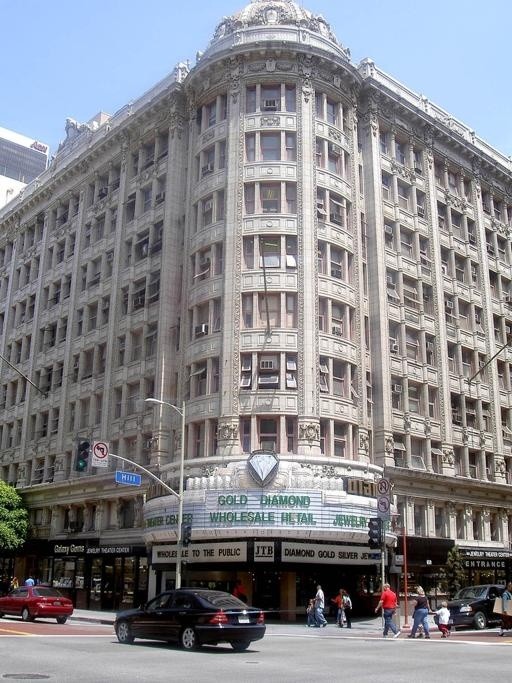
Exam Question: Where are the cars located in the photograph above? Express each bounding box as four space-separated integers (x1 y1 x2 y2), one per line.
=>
0 586 74 624
114 589 266 651
434 584 506 630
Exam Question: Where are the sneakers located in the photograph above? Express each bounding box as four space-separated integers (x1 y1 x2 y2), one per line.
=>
394 631 401 639
407 633 430 639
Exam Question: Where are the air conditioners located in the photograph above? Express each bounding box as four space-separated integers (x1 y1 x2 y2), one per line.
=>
388 344 397 352
391 383 402 393
444 300 454 309
262 97 277 110
384 224 392 234
194 324 208 334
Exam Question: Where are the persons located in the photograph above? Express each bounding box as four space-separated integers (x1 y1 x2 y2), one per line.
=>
498 581 512 637
304 583 353 629
374 581 451 641
8 574 35 590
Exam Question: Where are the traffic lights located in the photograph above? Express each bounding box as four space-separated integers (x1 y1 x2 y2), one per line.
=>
368 518 381 549
72 436 91 473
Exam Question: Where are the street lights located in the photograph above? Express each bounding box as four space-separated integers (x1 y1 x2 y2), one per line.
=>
144 398 186 590
